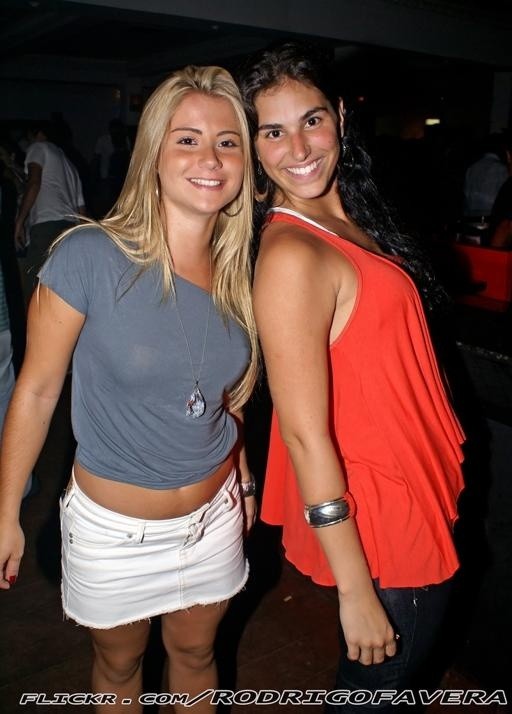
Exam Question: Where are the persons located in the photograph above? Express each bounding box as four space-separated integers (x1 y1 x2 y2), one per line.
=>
0 45 468 714
461 130 512 250
